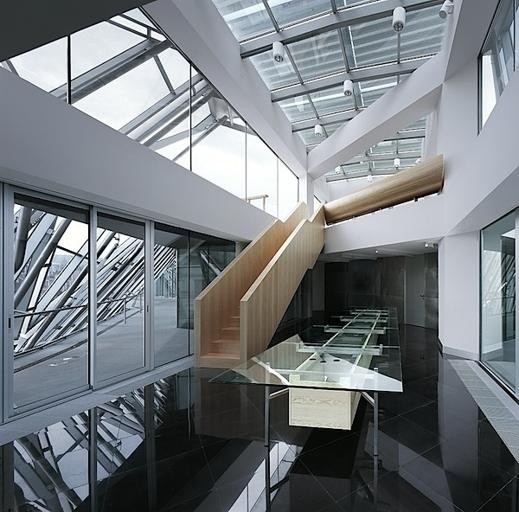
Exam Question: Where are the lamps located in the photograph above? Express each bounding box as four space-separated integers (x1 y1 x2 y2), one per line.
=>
393 158 400 169
344 79 353 96
439 0 454 19
273 42 284 63
392 7 405 32
315 125 322 135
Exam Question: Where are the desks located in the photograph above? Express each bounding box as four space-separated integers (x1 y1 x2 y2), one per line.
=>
209 304 405 457
